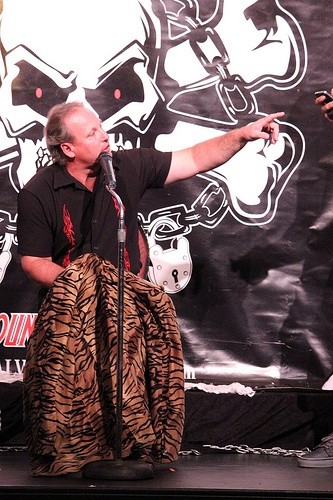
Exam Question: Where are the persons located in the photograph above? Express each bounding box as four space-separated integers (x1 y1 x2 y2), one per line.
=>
19 98 285 287
296 84 333 470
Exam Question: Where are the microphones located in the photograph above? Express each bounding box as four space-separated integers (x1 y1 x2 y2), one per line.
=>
99 152 119 190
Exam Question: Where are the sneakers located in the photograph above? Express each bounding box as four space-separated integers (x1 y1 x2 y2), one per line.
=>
297 434 333 467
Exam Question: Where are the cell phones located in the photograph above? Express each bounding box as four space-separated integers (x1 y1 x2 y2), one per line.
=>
314 90 333 120
